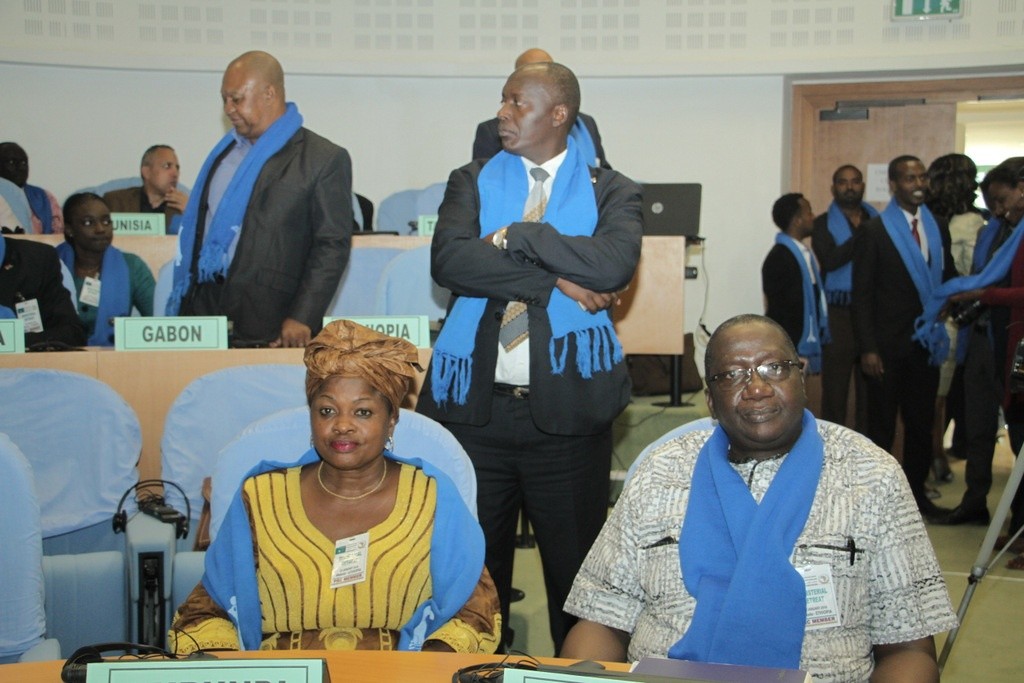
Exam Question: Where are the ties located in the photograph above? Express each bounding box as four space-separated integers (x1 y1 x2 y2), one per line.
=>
498 168 551 352
911 217 923 246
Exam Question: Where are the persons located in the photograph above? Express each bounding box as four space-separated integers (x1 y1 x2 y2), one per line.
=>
411 46 645 664
162 321 504 653
102 145 189 234
55 192 157 346
175 53 355 348
554 314 963 683
0 141 53 234
0 235 87 349
762 152 1024 571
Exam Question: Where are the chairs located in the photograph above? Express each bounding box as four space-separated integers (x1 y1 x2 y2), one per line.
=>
0 368 476 664
0 177 453 333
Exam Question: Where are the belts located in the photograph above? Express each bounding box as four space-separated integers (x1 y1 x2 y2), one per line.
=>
494 382 530 398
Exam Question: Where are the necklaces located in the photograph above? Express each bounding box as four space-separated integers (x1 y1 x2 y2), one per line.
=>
313 454 391 506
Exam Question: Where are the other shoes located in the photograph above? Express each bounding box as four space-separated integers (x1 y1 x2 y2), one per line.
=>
917 494 947 521
930 506 989 525
932 453 954 486
990 534 1024 553
509 588 525 604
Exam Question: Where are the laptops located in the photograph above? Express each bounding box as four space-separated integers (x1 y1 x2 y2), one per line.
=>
638 181 702 236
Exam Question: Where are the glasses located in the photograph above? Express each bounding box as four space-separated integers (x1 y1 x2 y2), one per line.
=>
710 361 806 393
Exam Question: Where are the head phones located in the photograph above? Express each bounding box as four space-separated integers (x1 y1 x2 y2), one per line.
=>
61 642 221 683
452 662 537 683
113 480 191 541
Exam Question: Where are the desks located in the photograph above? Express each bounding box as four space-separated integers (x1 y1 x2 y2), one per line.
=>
0 650 631 683
0 233 696 603
